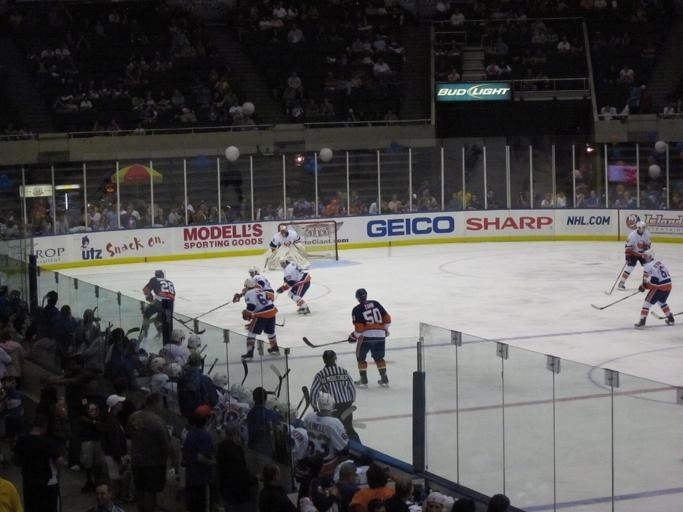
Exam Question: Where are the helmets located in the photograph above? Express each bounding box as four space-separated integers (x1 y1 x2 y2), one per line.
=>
168 329 186 345
186 334 203 350
247 266 259 273
315 392 336 411
150 356 166 372
279 224 287 231
277 256 289 264
354 288 367 300
421 491 455 510
214 371 299 423
164 361 184 380
242 278 257 288
154 269 164 278
256 461 281 481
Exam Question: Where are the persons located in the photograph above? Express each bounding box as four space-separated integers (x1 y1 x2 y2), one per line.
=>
238 266 274 295
136 267 176 351
346 288 391 386
309 349 361 445
264 240 310 270
240 278 280 358
1 286 280 511
3 0 681 241
268 224 305 253
280 422 511 512
275 256 311 313
302 391 349 477
633 248 675 327
617 219 656 289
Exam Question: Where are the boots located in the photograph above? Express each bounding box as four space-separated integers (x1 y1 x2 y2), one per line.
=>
295 305 310 314
353 376 369 385
375 374 389 385
240 349 254 358
618 282 677 328
266 345 280 353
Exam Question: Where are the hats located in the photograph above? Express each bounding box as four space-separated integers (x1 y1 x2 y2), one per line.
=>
338 461 360 479
187 351 204 365
322 349 337 363
194 403 216 417
104 393 126 408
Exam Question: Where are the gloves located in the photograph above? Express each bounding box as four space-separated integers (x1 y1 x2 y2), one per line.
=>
346 330 358 344
144 294 153 302
276 286 284 294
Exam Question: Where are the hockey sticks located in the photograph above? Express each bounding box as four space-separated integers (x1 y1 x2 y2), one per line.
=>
340 406 356 422
173 317 207 334
303 337 350 348
298 386 311 419
603 260 627 295
270 363 291 399
275 320 286 326
590 290 641 310
651 311 683 319
241 359 248 385
180 301 230 324
